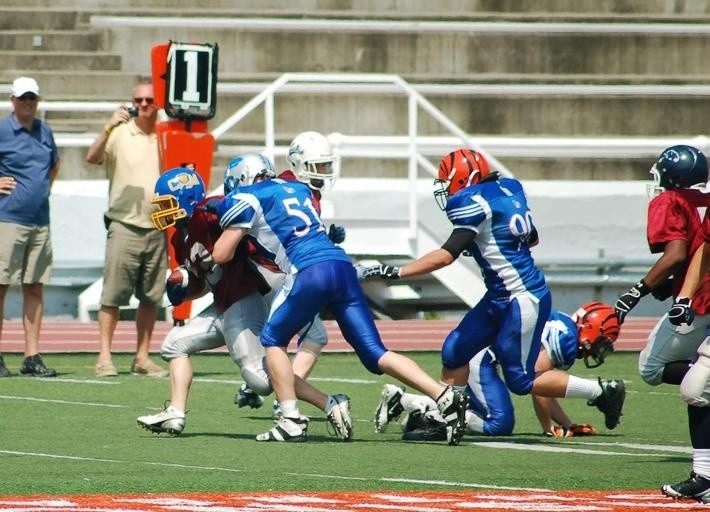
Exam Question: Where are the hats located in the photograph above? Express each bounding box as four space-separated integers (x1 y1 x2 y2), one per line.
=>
12 77 39 97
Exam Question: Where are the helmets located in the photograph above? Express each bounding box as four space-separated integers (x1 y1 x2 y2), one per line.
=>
438 150 490 193
646 145 708 197
571 302 620 360
150 132 335 230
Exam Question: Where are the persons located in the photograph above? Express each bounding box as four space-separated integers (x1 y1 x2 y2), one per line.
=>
137 166 353 442
233 131 346 425
200 154 470 447
354 148 626 443
373 300 622 442
86 74 173 376
668 198 708 424
614 145 710 503
0 78 66 379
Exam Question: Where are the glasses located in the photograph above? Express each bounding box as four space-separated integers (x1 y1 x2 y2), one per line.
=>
17 94 36 100
133 98 152 102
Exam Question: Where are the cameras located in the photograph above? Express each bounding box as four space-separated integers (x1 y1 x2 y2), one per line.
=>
128 107 139 116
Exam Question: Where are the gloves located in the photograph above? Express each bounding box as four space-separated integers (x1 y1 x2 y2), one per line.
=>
329 224 344 243
614 279 694 325
548 424 595 437
361 264 401 281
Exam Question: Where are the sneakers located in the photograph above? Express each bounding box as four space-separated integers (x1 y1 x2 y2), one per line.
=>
95 362 118 377
137 400 189 435
587 377 626 429
662 470 710 504
235 384 470 446
21 354 56 376
0 356 11 376
132 361 169 376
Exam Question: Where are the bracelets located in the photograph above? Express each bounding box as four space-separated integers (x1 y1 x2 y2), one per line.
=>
104 124 112 136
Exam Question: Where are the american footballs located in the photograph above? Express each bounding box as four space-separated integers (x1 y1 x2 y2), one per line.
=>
169 264 213 302
